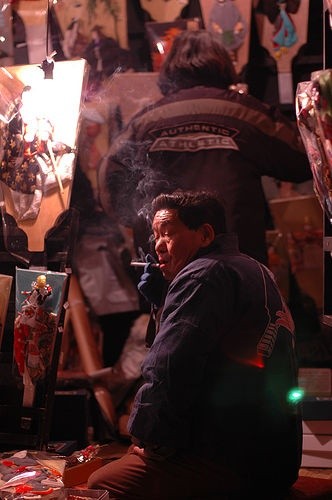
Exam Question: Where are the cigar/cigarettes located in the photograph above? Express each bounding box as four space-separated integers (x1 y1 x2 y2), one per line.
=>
131 261 160 266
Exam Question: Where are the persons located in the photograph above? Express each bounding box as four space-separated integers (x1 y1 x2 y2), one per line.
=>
87 189 303 500
97 29 313 347
